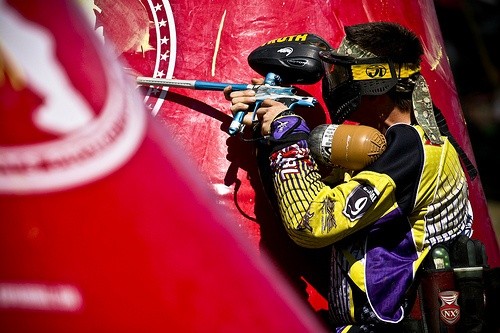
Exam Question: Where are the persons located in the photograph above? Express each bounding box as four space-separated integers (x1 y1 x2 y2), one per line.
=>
219 19 473 333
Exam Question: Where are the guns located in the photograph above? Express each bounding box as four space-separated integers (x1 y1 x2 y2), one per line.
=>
137 34 389 169
